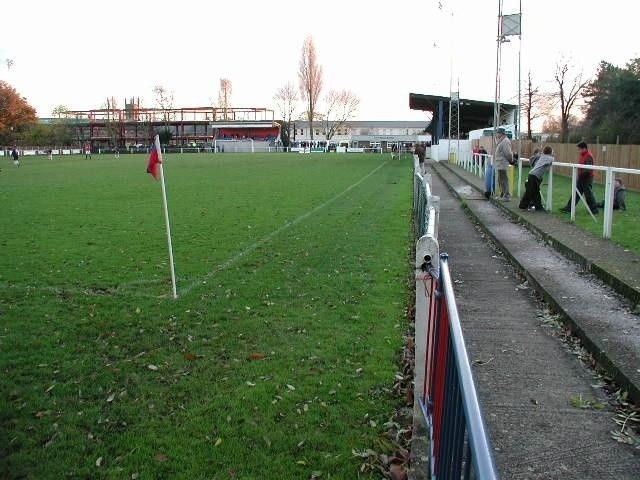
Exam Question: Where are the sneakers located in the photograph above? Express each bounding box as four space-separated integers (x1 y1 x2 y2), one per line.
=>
495 194 511 201
559 207 571 213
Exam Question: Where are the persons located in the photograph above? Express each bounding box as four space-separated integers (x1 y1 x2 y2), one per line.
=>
222 132 256 142
264 134 277 142
46 144 53 160
519 146 555 212
10 148 19 168
512 150 518 168
493 128 515 202
290 141 319 149
484 144 495 200
472 145 487 170
558 141 599 216
391 141 431 177
177 139 208 153
83 141 92 160
451 131 467 140
525 147 545 211
340 143 346 148
359 143 377 152
596 178 628 211
111 144 120 160
160 142 169 153
327 143 336 152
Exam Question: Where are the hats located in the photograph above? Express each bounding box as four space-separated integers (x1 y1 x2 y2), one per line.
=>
496 128 506 134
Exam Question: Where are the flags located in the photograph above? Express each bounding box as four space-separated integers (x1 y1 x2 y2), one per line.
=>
145 144 162 182
